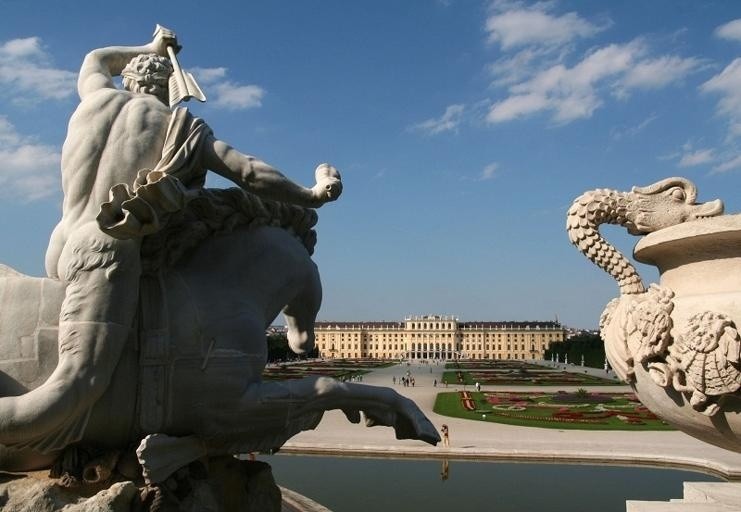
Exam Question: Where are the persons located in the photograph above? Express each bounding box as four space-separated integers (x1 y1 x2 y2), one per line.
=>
440 460 448 482
337 353 481 391
0 28 343 455
441 424 449 445
